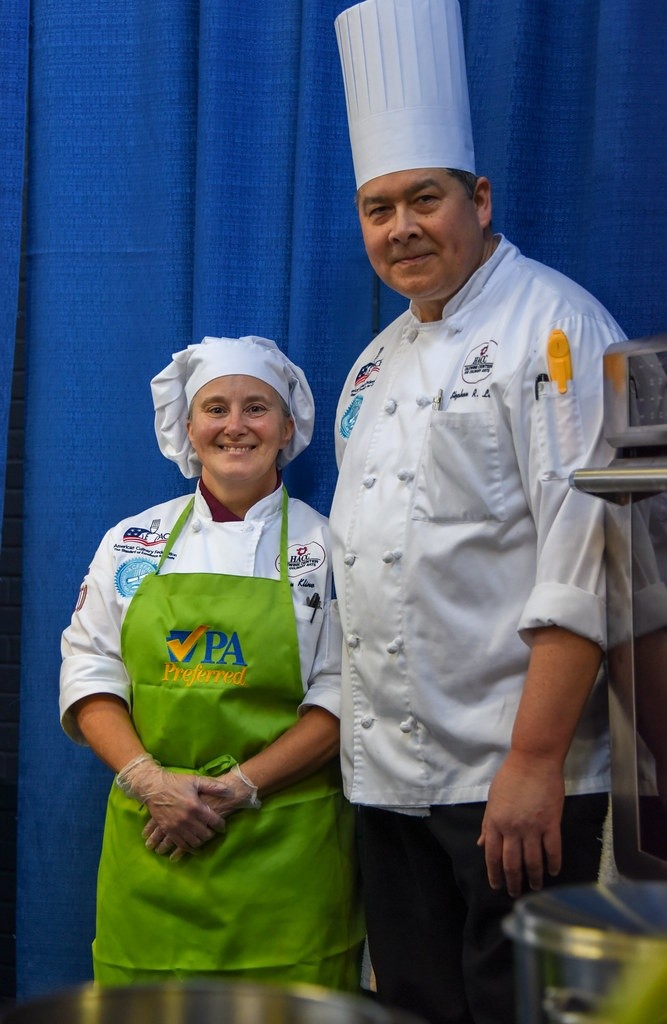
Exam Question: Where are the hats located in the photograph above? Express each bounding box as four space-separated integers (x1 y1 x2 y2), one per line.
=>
150 335 316 480
334 0 476 190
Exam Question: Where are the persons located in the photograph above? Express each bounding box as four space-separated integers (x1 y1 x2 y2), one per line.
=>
59 335 367 991
605 493 667 883
325 126 631 1024
